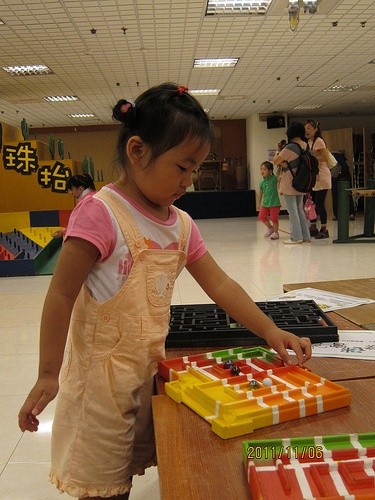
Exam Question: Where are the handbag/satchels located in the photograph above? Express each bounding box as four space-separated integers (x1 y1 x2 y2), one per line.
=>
326 148 338 169
303 193 318 221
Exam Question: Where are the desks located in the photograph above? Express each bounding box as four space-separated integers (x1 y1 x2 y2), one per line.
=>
195 159 222 191
149 279 375 500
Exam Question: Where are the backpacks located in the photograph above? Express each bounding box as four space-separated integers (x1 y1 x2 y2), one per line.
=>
283 141 319 193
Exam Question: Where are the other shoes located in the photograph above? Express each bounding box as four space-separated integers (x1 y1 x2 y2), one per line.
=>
265 226 275 237
270 232 280 239
315 229 329 239
284 237 303 244
303 239 311 243
308 226 319 236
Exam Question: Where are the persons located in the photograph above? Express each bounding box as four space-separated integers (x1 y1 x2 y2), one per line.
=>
17 83 312 500
257 119 332 244
51 173 97 238
